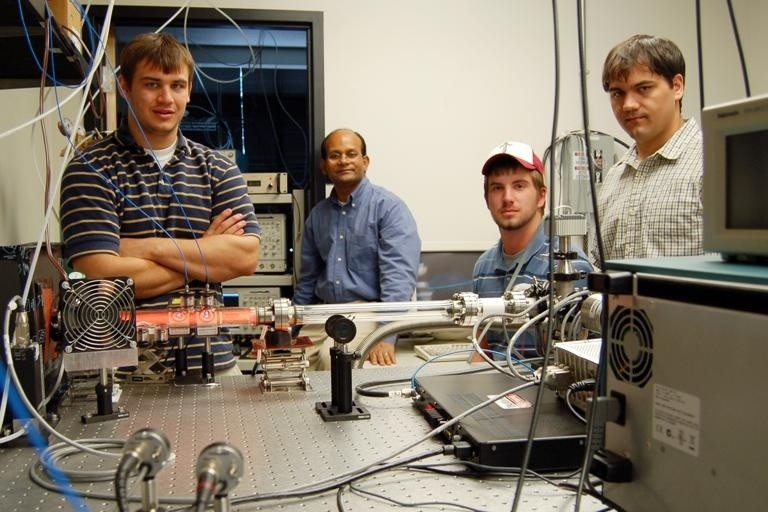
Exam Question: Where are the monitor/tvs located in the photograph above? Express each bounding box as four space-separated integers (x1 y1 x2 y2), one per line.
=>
701 95 768 258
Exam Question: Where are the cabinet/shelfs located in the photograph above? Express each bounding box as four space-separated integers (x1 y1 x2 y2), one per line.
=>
221 188 304 305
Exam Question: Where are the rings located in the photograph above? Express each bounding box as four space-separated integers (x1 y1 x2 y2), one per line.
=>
384 351 389 357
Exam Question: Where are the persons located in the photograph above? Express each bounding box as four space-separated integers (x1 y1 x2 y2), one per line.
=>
288 129 423 367
58 30 262 380
466 141 603 363
588 34 704 268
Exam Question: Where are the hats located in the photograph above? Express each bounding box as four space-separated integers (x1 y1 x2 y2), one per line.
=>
481 139 545 181
